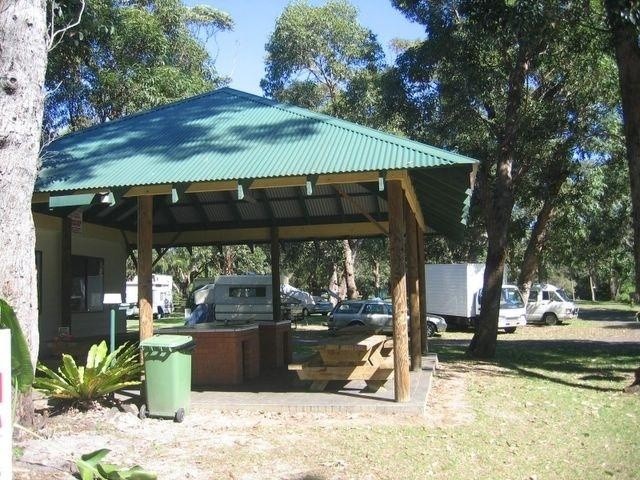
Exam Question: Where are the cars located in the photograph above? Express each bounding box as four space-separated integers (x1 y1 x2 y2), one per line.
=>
291 295 333 317
328 300 447 337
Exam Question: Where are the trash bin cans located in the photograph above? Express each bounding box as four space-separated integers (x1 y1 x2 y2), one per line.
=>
138 334 193 422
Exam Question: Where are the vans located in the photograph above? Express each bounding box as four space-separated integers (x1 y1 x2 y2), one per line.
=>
508 283 580 326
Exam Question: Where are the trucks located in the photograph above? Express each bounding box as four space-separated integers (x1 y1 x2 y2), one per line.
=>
425 263 527 334
125 273 173 319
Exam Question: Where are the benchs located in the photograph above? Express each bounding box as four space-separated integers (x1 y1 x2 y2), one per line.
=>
288 340 394 393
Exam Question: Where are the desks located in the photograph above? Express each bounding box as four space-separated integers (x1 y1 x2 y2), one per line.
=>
308 333 388 396
